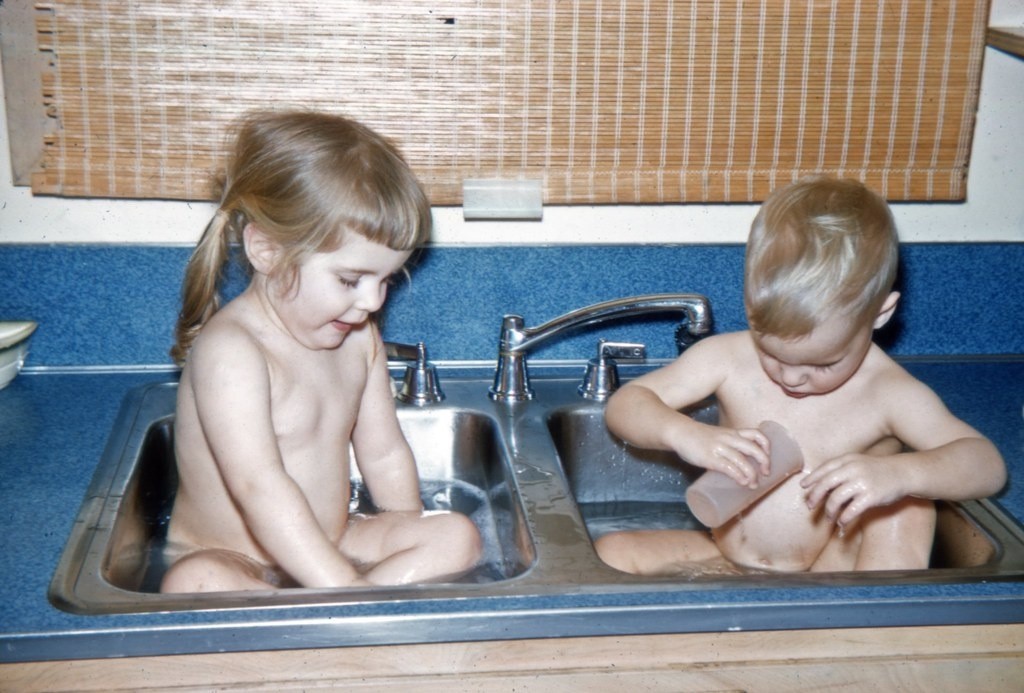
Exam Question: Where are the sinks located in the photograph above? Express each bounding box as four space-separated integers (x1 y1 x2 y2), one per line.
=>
540 379 1024 591
48 380 538 616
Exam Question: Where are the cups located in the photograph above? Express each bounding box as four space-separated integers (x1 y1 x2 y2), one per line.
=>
685 421 803 528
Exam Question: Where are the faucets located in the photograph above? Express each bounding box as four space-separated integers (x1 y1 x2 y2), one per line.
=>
488 294 712 402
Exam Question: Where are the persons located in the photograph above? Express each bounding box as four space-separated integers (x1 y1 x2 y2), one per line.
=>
595 176 1007 575
158 110 484 597
